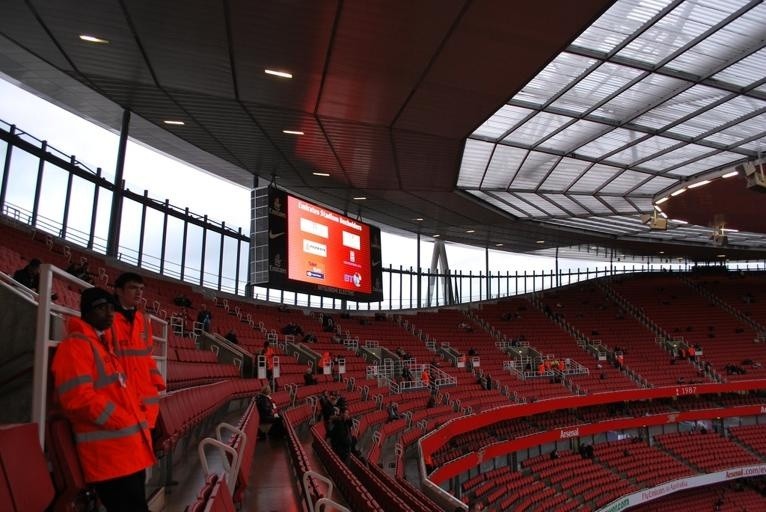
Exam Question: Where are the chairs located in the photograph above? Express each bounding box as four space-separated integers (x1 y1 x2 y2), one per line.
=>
0 214 766 510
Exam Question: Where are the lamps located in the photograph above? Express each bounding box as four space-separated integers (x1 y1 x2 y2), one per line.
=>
736 151 766 194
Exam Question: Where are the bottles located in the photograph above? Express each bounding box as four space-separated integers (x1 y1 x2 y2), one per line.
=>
79 287 115 312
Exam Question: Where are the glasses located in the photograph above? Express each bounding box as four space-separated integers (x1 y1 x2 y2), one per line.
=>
736 151 766 194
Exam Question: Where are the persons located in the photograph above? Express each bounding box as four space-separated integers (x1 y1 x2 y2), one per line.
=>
0 257 766 460
50 284 157 511
